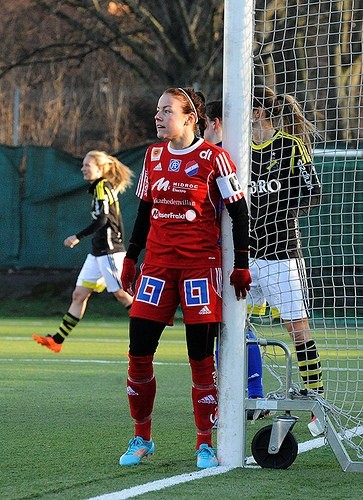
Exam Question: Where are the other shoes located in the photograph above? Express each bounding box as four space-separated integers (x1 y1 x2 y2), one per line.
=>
247 396 270 421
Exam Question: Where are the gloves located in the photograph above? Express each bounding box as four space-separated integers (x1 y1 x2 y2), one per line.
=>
120 257 137 296
229 268 252 302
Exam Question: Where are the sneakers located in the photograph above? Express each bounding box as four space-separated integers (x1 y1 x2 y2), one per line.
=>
195 443 219 469
31 333 63 354
118 436 156 466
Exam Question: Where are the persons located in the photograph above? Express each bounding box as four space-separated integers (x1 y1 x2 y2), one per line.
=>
245 85 325 422
32 151 133 353
119 87 254 467
200 100 271 429
